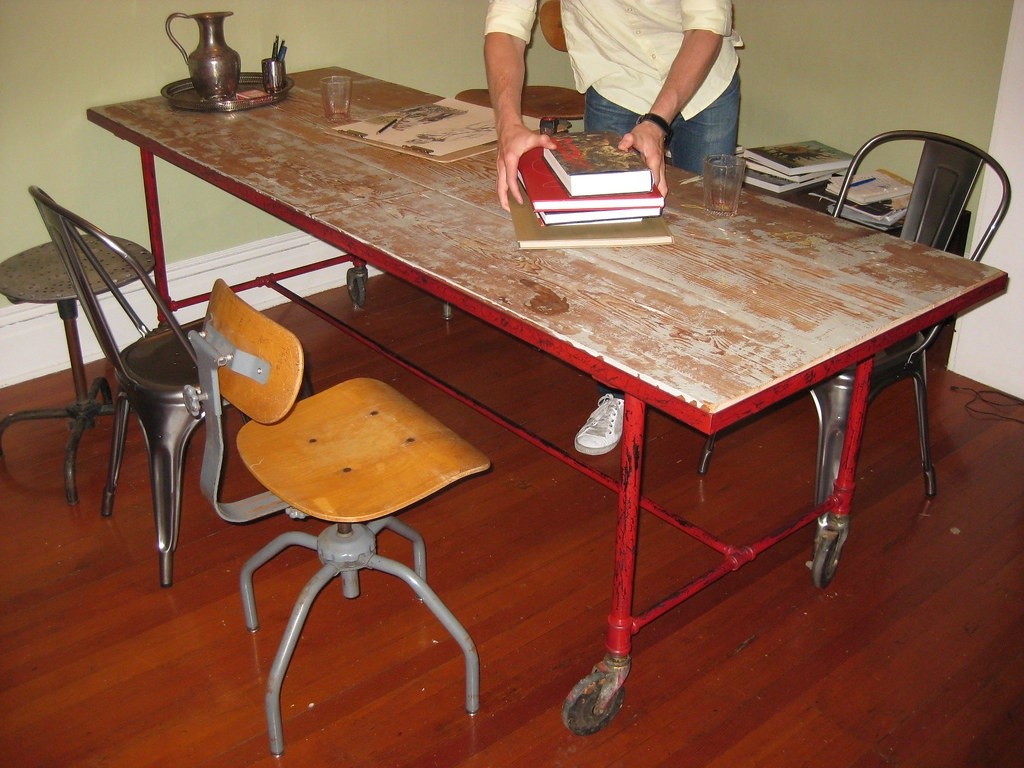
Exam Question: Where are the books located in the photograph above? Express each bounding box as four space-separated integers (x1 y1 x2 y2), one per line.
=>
734 140 914 231
506 129 675 250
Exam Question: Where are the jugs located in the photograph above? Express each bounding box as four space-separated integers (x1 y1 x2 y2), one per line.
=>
164 11 240 101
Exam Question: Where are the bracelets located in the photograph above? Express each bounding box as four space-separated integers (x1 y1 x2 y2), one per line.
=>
636 113 673 147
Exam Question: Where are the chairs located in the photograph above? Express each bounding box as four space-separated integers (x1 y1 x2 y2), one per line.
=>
455 0 585 133
693 128 1011 502
26 184 314 589
186 280 491 756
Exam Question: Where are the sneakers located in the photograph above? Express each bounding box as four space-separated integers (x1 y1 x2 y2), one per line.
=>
574 393 624 455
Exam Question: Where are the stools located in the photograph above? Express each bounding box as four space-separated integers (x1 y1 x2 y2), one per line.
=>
0 235 157 505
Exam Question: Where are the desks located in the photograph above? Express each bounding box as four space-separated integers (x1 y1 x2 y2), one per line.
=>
86 68 1009 736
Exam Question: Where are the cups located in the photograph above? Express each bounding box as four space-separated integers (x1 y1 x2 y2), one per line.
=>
704 153 746 216
261 58 285 94
320 76 351 123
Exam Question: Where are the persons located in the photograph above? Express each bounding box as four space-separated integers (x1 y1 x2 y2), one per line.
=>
484 0 741 456
